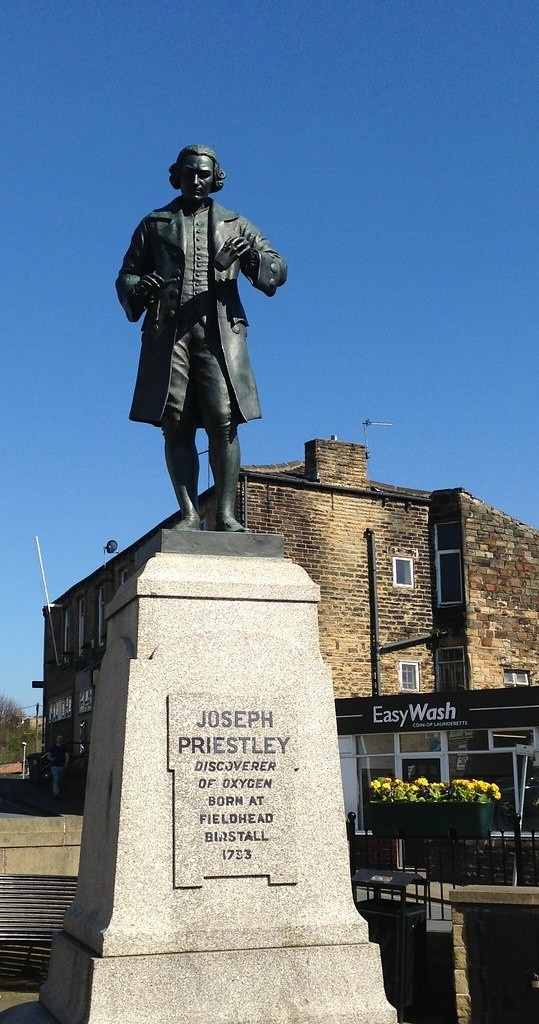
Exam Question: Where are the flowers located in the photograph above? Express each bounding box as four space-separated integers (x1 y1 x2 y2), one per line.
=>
369 777 500 804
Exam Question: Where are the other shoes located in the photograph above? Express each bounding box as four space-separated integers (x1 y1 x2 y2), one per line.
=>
54 794 59 800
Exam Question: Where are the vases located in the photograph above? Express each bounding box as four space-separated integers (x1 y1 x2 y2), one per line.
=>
373 802 496 839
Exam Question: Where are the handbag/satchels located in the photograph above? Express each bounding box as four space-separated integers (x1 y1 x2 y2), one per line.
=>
47 755 56 764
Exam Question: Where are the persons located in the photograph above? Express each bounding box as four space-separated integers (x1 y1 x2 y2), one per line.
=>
47 735 70 801
113 144 289 533
